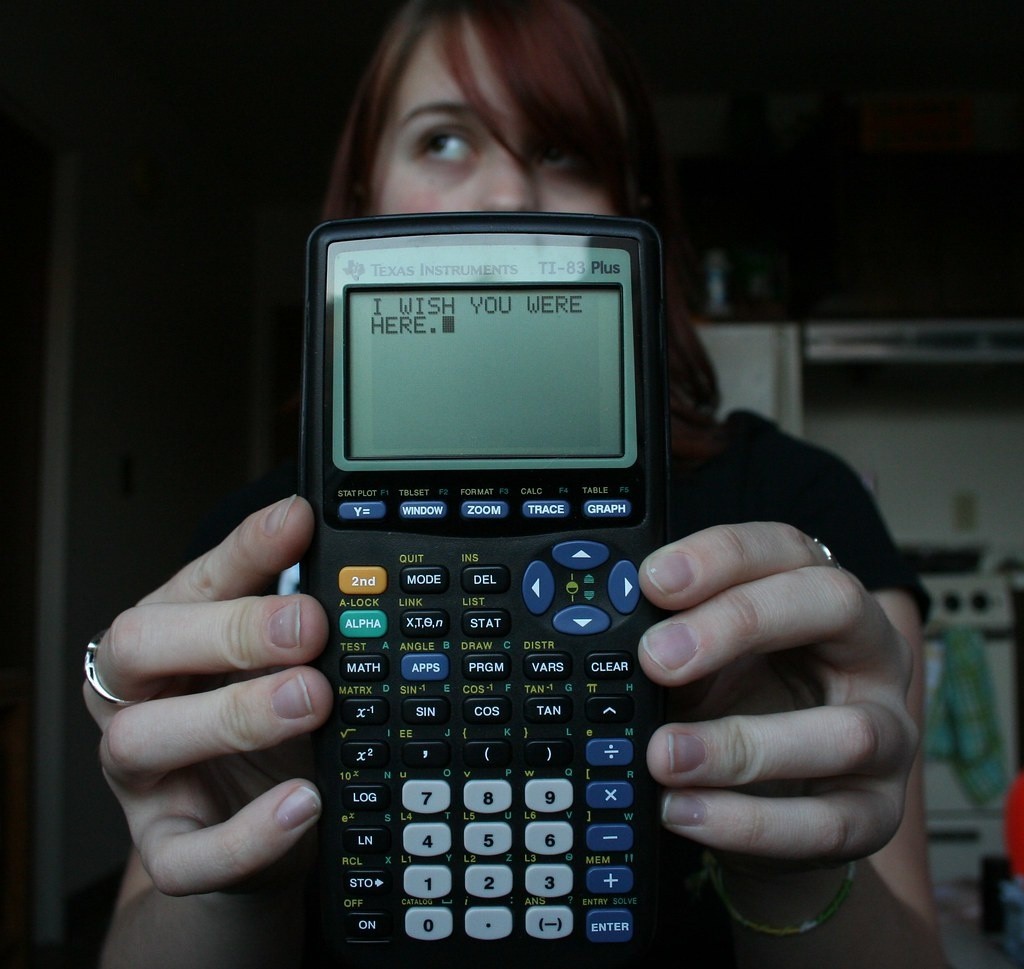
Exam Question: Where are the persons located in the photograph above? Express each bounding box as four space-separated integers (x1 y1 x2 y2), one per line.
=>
82 0 944 969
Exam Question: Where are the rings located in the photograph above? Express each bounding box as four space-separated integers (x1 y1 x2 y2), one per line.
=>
81 626 162 706
812 535 840 570
